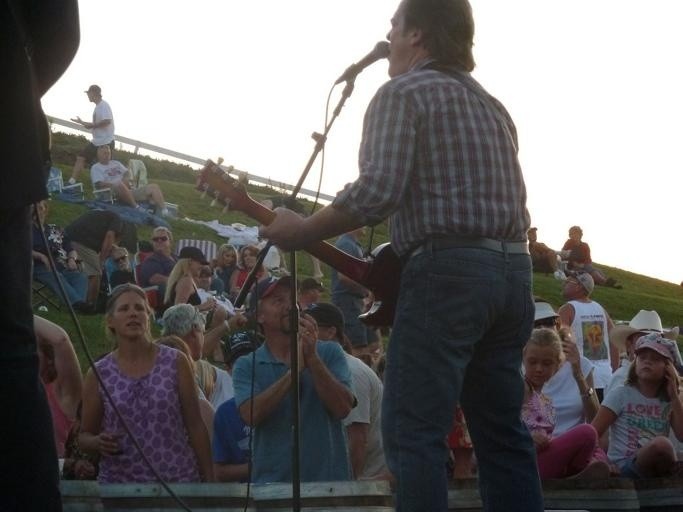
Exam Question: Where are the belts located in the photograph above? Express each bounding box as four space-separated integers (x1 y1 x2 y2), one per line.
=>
410 234 530 257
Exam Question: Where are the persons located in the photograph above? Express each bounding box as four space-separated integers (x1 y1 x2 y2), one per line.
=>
255 0 544 512
0 0 83 512
63 85 115 186
21 200 682 511
89 145 179 220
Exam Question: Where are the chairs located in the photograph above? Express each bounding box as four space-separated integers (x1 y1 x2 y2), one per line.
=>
47 168 85 202
31 259 62 311
92 184 117 205
132 250 163 320
104 255 118 296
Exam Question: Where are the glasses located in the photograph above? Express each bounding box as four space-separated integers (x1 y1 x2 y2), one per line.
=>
114 254 128 262
152 236 168 242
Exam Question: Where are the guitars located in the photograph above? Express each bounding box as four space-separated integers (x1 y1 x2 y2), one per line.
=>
195 157 407 326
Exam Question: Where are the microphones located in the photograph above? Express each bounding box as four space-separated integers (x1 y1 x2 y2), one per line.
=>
335 39 390 85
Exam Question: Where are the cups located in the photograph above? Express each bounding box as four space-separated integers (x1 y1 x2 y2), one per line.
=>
105 431 130 455
206 296 215 311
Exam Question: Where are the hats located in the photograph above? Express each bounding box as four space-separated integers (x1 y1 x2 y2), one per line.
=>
562 264 595 293
634 333 677 361
609 309 678 349
304 302 345 331
248 274 297 314
300 277 324 292
84 84 102 94
178 246 210 265
226 331 254 356
533 298 560 323
